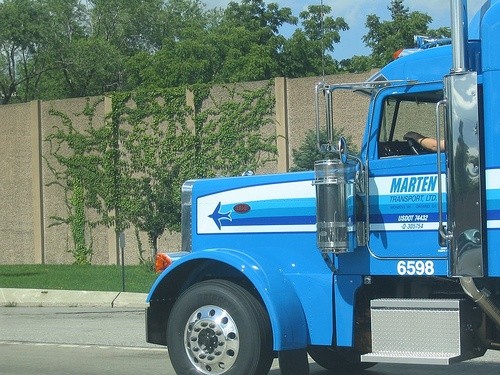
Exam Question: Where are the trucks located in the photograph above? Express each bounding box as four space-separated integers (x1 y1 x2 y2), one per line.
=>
144 1 499 375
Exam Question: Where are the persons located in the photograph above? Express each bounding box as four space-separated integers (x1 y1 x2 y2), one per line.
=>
401 127 446 153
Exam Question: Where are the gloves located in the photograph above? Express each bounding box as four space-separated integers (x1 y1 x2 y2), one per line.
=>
403 131 423 141
